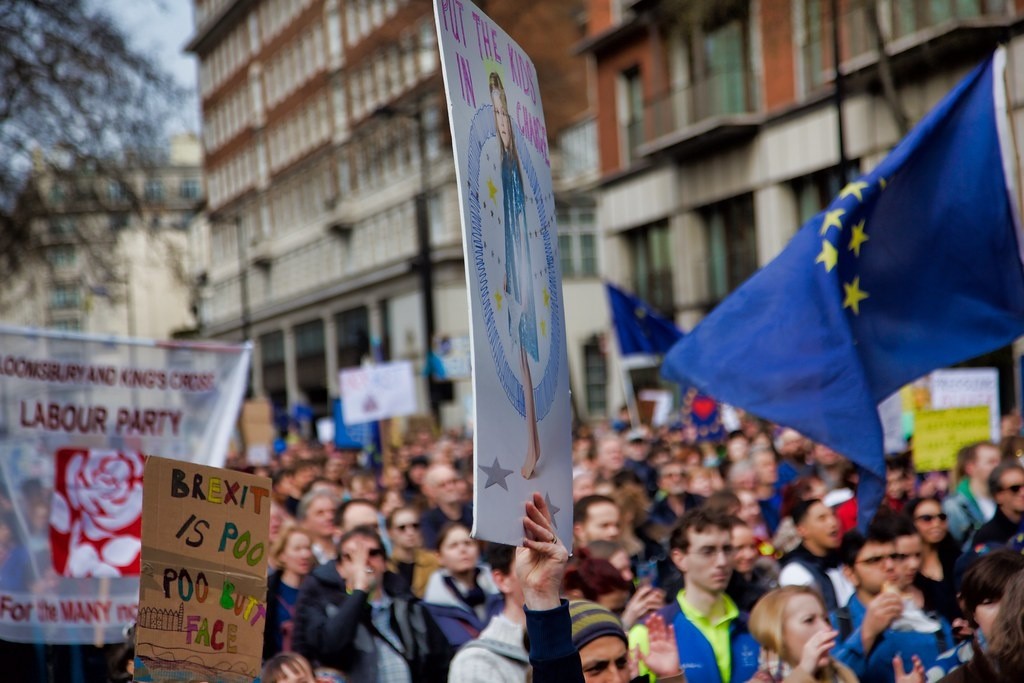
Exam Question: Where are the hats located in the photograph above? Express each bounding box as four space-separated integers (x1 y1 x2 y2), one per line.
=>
628 430 648 444
569 597 630 656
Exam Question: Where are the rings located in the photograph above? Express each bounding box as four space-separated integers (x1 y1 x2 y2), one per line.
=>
549 531 557 544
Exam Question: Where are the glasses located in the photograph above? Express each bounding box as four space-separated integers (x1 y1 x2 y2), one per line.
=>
997 483 1024 492
853 553 897 567
391 523 419 531
685 546 734 557
897 551 924 563
341 549 381 562
913 513 946 522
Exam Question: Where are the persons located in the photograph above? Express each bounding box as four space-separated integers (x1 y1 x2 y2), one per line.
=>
491 70 542 479
1 403 1024 683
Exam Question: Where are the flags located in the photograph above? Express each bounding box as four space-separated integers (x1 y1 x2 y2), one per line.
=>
609 282 687 357
659 44 1024 535
